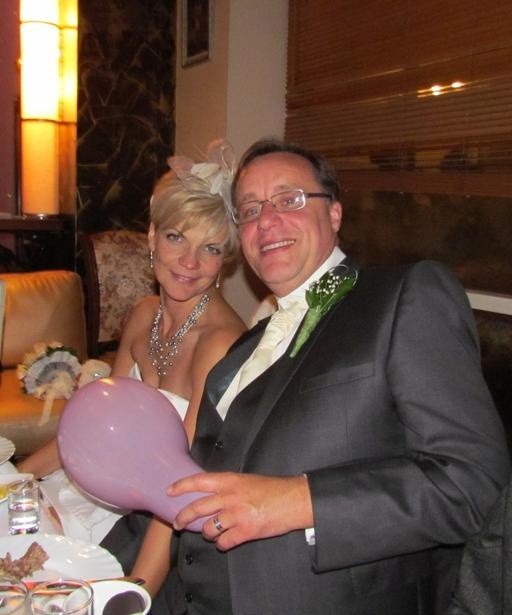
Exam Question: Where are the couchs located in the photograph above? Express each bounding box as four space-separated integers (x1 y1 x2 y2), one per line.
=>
79 226 512 440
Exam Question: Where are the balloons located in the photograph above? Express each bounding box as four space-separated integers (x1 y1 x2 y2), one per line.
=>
57 374 220 535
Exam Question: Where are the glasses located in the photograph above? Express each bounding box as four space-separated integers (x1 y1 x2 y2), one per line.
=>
230 190 333 226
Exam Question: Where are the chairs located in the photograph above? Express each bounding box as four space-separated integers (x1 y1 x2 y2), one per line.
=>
0 271 105 458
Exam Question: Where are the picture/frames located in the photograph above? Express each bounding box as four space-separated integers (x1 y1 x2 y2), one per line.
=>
182 0 213 68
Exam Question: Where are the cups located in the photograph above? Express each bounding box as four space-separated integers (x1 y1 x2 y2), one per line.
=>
7 479 40 536
0 575 94 615
41 580 151 615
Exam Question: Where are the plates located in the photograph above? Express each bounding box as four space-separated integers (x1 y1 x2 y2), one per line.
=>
0 436 16 464
0 534 125 583
0 474 33 503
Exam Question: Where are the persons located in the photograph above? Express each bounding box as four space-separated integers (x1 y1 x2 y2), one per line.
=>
8 157 249 548
446 483 511 614
147 137 511 615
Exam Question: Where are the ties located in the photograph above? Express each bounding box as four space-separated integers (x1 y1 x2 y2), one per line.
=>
234 301 306 392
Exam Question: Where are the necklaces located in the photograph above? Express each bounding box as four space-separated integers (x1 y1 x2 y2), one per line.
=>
149 290 214 381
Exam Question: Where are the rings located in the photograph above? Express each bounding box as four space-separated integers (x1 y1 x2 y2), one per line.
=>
212 514 226 535
